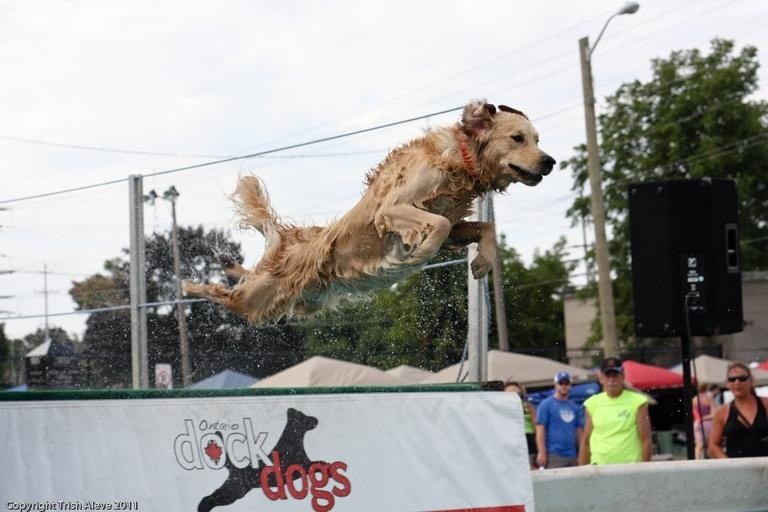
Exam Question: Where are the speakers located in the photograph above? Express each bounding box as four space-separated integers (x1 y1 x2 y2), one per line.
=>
627 180 743 339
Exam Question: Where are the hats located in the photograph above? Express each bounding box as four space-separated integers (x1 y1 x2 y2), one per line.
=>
552 370 573 385
598 357 623 374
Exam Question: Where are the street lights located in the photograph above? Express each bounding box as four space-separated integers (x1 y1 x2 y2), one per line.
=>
576 0 644 364
162 181 193 385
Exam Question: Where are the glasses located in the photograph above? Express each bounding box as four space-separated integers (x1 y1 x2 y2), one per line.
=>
725 375 750 383
558 381 569 386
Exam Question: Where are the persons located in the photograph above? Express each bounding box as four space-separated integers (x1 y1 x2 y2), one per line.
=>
706 364 768 459
576 355 653 465
533 369 585 468
691 382 708 459
523 398 536 469
502 381 525 405
700 382 721 440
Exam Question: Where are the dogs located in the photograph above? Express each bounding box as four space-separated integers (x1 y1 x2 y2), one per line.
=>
180 97 557 330
